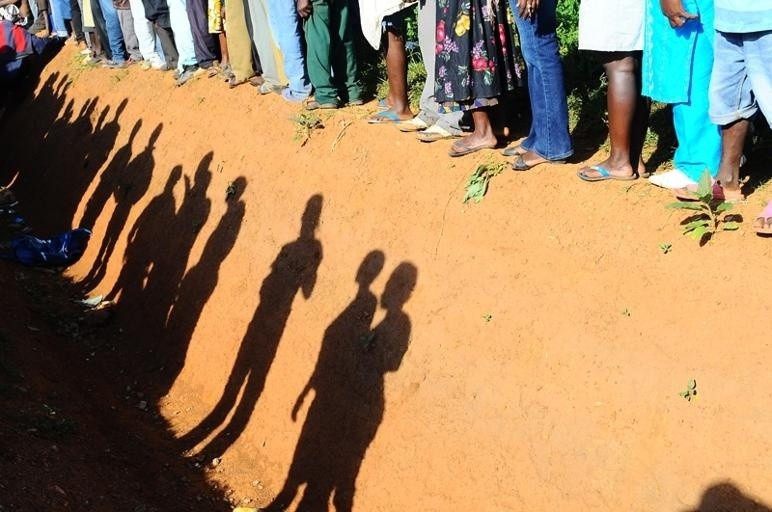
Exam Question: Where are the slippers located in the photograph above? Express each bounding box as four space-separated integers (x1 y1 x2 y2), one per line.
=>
753 201 772 234
368 100 499 157
579 164 650 181
501 146 567 171
677 184 745 201
78 40 363 110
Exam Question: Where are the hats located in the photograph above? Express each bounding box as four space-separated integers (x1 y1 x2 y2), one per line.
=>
4 4 19 22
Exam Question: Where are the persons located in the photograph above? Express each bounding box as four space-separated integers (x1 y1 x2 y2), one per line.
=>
0 2 575 170
575 2 772 236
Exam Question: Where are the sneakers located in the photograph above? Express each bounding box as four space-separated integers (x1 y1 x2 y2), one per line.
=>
648 169 698 190
